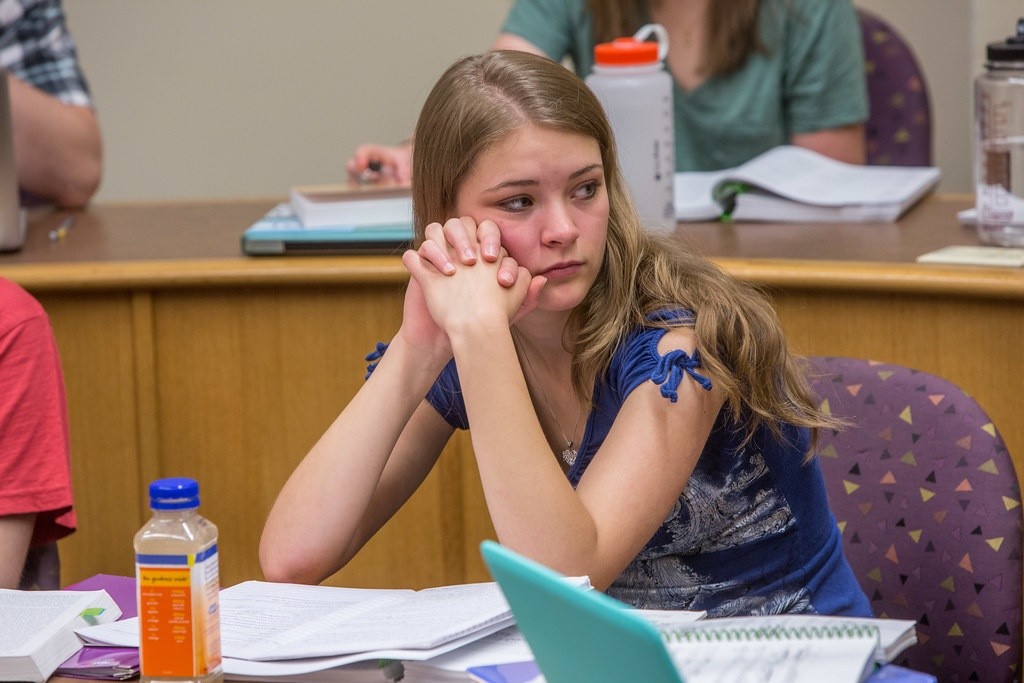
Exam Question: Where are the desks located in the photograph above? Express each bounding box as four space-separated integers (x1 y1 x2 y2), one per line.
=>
2 185 1024 590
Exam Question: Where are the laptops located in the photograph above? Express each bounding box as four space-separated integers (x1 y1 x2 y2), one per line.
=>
240 202 415 255
479 541 685 683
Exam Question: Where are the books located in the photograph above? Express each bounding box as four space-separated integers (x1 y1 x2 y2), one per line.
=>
218 576 917 683
672 143 943 221
0 588 123 683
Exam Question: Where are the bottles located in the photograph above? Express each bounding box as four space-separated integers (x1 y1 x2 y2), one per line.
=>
132 477 224 683
582 22 678 239
973 17 1024 250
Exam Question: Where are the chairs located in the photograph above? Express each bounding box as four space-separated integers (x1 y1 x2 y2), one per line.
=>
792 356 1023 683
853 5 932 168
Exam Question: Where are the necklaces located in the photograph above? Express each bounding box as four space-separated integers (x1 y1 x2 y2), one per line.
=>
513 322 582 466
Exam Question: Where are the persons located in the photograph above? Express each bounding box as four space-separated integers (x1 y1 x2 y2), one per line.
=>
0 0 103 209
343 0 869 190
258 51 875 619
0 267 78 591
